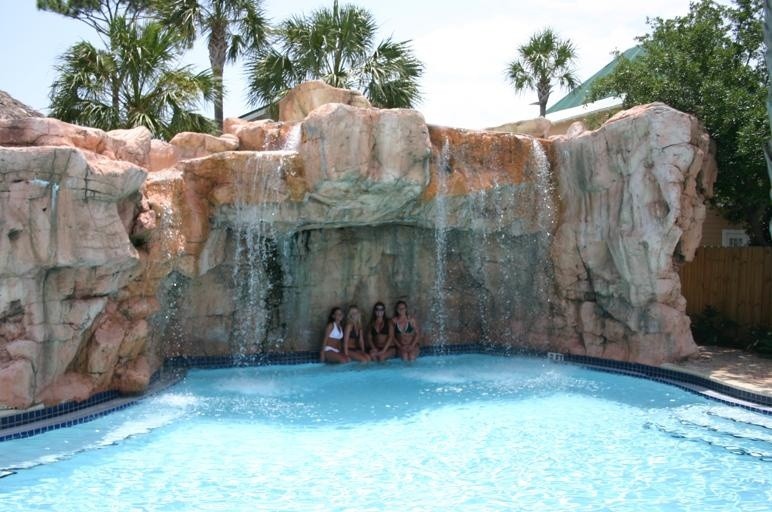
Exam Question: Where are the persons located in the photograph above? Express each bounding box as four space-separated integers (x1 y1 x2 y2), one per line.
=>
321 301 420 363
